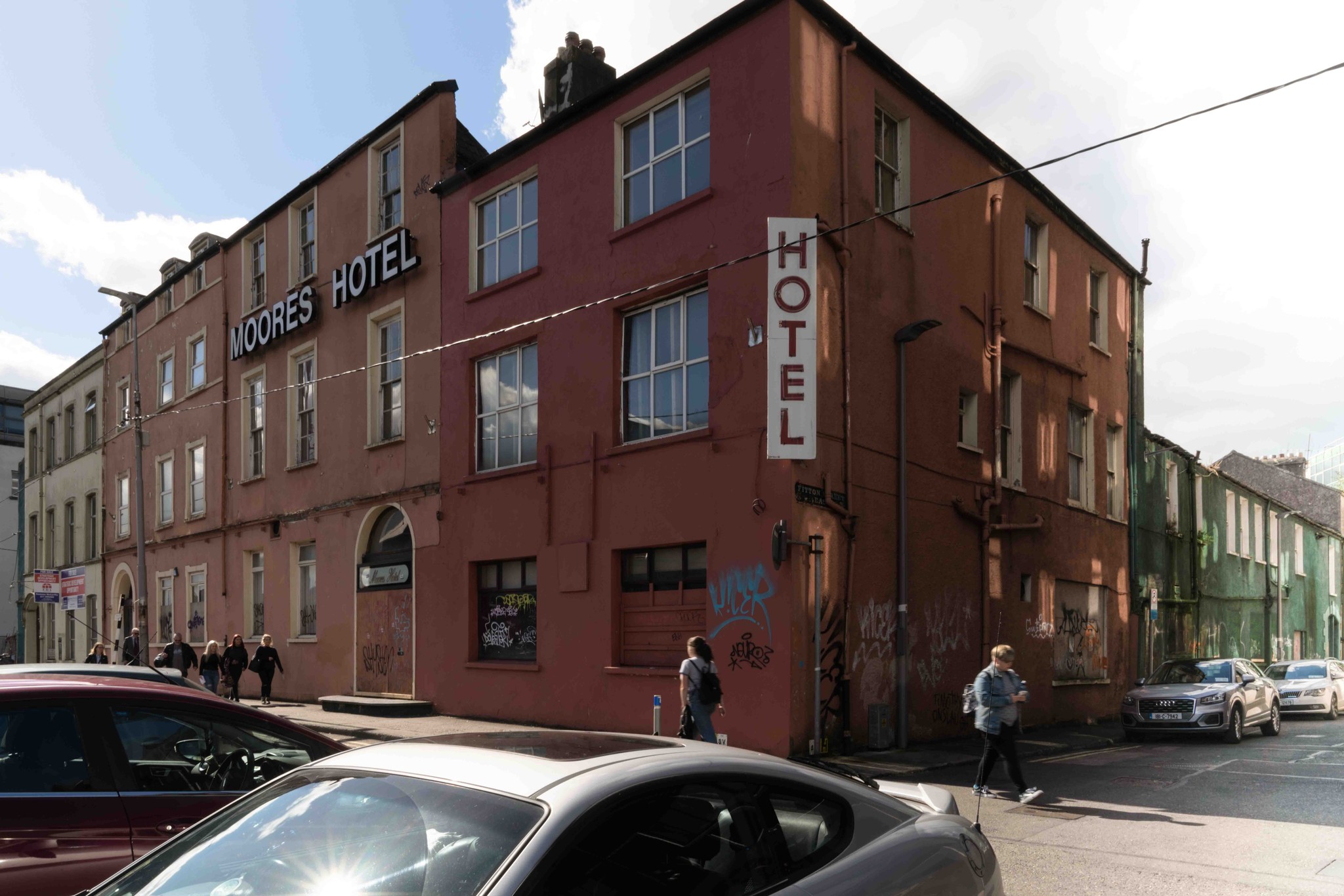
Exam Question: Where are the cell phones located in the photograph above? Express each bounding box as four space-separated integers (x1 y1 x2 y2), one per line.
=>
1019 691 1027 695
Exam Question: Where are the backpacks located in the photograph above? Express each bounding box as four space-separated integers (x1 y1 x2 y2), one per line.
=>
963 684 978 714
687 659 723 705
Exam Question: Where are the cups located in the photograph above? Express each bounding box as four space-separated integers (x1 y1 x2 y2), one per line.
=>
1018 691 1028 701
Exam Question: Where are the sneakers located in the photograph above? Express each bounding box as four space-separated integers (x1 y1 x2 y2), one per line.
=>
1019 788 1043 804
972 786 997 798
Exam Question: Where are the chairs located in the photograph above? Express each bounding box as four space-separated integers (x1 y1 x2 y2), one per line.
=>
655 795 721 896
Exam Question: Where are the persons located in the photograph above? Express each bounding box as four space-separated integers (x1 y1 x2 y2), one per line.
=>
972 644 1044 805
199 634 248 702
680 636 726 744
255 634 284 704
84 642 108 664
162 632 198 678
122 628 140 666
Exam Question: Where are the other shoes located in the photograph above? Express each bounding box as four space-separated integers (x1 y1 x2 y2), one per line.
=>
261 696 271 704
229 697 239 702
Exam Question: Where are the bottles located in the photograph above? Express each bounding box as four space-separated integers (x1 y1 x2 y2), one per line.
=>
1018 680 1027 691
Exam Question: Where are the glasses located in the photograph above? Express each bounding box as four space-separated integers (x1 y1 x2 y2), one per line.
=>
174 638 182 642
134 633 139 635
999 658 1015 663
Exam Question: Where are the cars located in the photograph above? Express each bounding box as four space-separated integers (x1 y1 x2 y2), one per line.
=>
0 658 353 896
1262 657 1344 720
82 728 1005 896
1119 657 1281 744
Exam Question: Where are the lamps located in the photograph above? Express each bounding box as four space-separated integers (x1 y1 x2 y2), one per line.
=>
1315 532 1326 540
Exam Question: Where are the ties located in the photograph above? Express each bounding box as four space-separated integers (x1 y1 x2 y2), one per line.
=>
134 638 137 648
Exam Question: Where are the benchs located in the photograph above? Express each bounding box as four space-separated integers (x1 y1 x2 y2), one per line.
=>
688 799 829 878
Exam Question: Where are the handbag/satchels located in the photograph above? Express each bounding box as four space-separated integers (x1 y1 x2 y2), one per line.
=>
221 657 233 687
248 645 261 672
154 653 166 668
